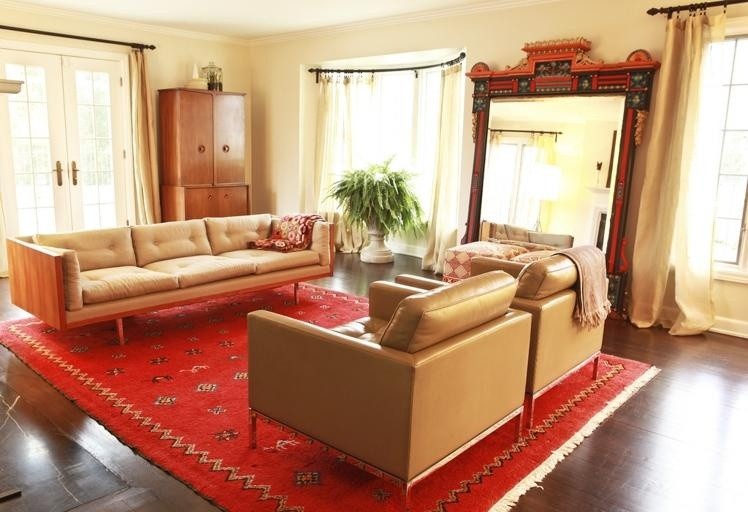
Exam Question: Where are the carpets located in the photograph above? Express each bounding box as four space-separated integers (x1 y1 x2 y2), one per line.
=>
1 281 658 512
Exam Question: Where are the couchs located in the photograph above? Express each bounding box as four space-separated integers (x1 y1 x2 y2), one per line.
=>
5 213 335 347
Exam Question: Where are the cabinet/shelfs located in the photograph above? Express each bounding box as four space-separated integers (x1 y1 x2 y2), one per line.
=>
158 88 252 221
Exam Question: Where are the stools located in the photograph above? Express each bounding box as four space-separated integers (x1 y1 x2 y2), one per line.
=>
444 240 526 283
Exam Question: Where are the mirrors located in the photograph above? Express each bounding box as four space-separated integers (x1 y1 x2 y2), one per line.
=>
460 37 662 328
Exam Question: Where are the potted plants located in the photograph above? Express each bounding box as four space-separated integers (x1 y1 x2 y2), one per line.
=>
321 159 429 264
